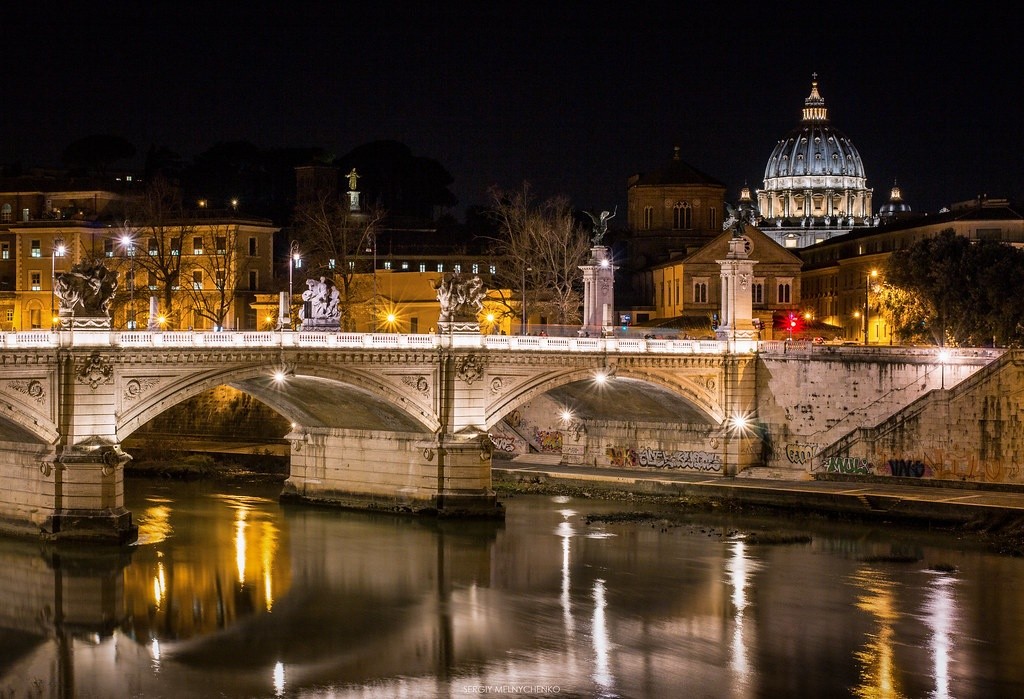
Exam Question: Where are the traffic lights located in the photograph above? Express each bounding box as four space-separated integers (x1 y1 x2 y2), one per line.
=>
790 317 796 327
789 318 796 329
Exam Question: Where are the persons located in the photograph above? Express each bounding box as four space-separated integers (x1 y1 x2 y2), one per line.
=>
501 329 550 341
644 334 656 339
683 334 690 340
724 201 755 237
54 258 120 312
737 273 754 285
776 214 881 227
582 205 617 246
436 273 485 316
585 332 589 338
302 277 340 318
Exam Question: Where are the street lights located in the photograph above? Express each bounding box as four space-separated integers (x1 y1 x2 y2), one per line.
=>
602 246 615 330
864 269 879 344
855 305 865 344
290 240 299 328
123 220 136 330
51 229 66 330
